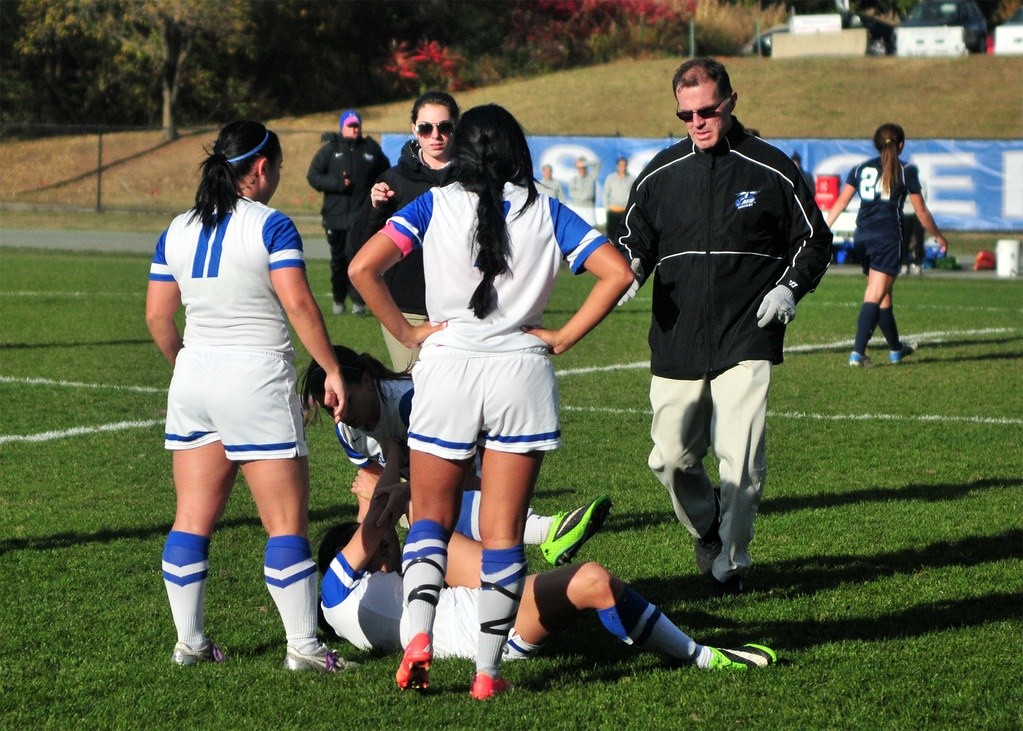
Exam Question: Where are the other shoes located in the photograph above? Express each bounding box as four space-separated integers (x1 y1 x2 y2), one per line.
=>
352 303 366 314
331 301 345 314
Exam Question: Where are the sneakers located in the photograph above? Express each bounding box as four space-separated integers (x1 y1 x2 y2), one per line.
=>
705 644 777 671
171 637 223 666
888 339 919 363
695 487 724 575
847 350 872 366
470 673 509 700
541 495 614 566
396 633 433 691
283 643 359 672
710 579 749 600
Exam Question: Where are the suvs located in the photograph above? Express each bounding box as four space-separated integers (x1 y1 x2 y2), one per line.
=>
890 0 987 55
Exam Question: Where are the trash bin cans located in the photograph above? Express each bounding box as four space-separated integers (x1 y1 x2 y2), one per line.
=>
996 239 1023 277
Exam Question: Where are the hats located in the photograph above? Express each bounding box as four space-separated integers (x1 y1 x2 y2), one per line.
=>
341 110 363 131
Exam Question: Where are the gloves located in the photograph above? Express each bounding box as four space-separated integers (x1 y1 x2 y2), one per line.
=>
757 285 797 328
618 258 644 307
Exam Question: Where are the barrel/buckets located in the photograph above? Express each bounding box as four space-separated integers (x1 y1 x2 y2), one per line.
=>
995 238 1019 277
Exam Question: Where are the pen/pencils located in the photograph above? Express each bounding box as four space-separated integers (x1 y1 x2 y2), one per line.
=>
383 188 387 193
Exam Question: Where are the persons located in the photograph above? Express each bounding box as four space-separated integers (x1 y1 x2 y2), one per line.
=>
746 128 760 136
348 100 635 696
569 157 597 231
317 438 776 672
146 122 371 672
306 108 392 315
825 123 948 366
537 166 566 206
296 344 414 579
612 56 835 593
361 91 463 365
792 152 816 197
602 157 636 242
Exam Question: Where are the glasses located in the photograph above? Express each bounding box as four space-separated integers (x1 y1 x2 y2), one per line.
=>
414 120 454 138
676 94 732 124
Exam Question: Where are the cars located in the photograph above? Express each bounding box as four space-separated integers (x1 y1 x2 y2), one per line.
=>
753 11 895 57
